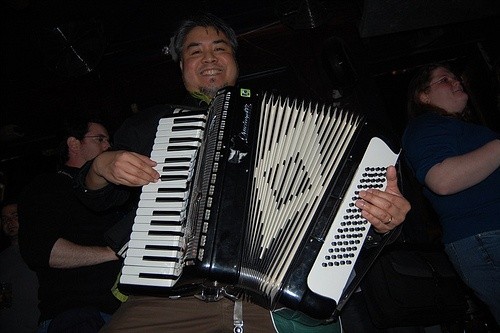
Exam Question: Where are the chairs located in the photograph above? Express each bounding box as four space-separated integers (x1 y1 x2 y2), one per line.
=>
362 258 465 333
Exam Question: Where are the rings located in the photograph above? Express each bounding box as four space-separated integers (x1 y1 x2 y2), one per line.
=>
384 217 392 224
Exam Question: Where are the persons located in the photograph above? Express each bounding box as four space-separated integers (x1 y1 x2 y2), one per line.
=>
0 205 426 333
401 63 500 320
18 115 139 317
74 10 411 333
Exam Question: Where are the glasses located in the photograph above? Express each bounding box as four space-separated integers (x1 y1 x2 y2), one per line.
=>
83 135 115 145
